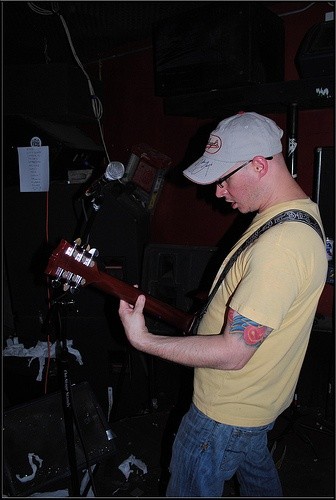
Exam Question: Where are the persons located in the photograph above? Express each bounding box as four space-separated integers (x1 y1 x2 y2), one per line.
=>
117 111 328 496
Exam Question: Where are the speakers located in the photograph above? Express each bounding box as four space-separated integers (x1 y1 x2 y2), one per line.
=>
152 1 285 97
2 382 118 497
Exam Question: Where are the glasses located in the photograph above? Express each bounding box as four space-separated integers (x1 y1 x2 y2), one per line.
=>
216 156 273 188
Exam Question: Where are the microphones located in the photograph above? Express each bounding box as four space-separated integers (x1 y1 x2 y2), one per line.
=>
83 161 125 198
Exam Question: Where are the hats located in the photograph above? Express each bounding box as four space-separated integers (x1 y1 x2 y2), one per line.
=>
183 112 283 185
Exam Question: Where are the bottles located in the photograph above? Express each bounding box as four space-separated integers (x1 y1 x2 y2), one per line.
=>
325 236 335 284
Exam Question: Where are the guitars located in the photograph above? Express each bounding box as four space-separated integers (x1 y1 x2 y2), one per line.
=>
44 237 194 329
